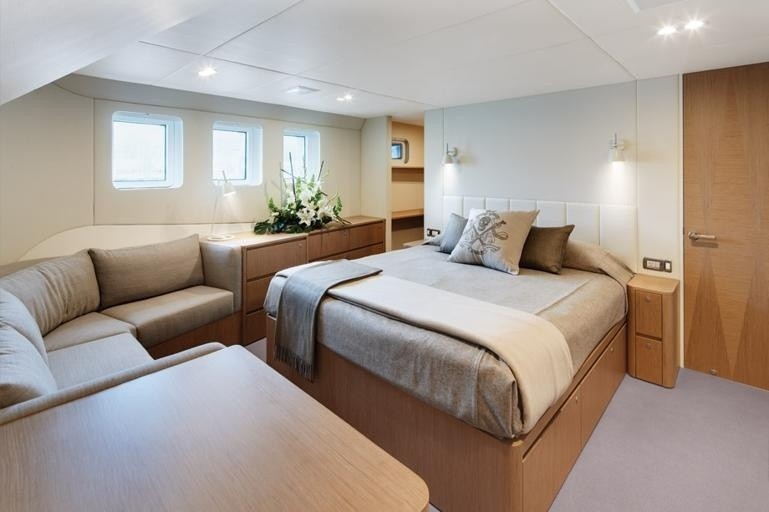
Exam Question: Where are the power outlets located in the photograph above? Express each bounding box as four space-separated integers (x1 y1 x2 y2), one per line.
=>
643 257 664 272
430 230 440 236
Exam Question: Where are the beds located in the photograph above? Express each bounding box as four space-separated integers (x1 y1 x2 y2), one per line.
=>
264 196 635 512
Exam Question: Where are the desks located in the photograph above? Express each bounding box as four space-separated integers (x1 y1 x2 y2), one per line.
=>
1 345 430 512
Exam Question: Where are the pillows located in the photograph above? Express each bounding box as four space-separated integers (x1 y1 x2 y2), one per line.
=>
0 323 58 408
89 233 205 309
421 207 635 313
0 248 100 335
0 288 48 364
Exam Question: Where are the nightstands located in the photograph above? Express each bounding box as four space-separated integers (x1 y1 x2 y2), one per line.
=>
402 239 425 247
626 273 680 388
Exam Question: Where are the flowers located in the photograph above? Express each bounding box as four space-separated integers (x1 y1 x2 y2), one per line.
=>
253 153 351 234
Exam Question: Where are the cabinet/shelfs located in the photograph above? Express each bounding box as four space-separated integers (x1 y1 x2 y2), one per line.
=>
242 235 307 346
307 220 386 263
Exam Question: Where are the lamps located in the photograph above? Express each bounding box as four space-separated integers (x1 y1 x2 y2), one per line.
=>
608 132 625 164
208 170 239 241
443 142 457 165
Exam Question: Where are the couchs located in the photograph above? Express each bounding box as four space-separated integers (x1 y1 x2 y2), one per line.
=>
0 240 242 424
